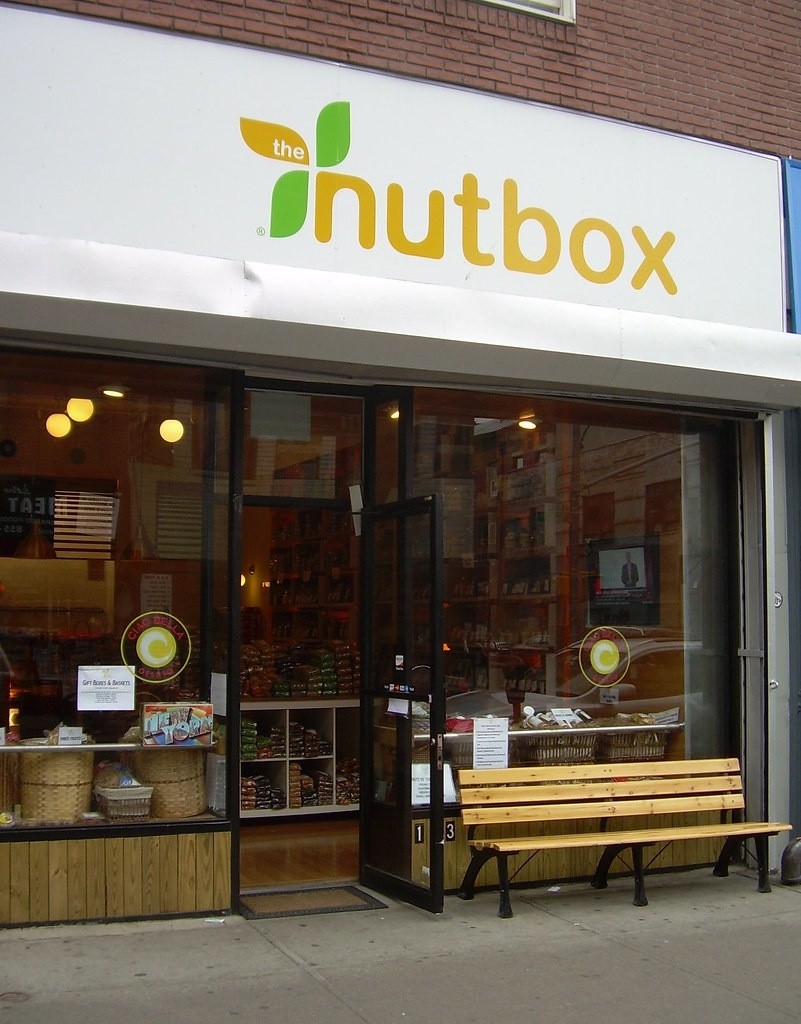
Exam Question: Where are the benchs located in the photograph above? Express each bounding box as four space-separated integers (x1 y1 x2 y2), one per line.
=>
456 757 793 918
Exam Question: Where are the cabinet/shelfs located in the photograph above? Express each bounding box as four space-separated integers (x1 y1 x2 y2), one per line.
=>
238 695 360 819
269 419 578 697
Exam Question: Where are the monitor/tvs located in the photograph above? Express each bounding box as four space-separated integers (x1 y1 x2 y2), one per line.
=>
587 532 662 626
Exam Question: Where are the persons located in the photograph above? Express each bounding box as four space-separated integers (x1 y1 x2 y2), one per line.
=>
622 551 639 584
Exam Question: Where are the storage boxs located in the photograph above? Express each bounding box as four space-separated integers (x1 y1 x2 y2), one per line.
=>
445 728 523 767
523 723 599 763
599 730 667 762
93 783 154 822
410 734 430 764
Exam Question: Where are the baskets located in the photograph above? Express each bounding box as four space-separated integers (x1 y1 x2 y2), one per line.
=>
405 730 671 771
91 783 153 823
121 748 208 819
16 735 95 821
